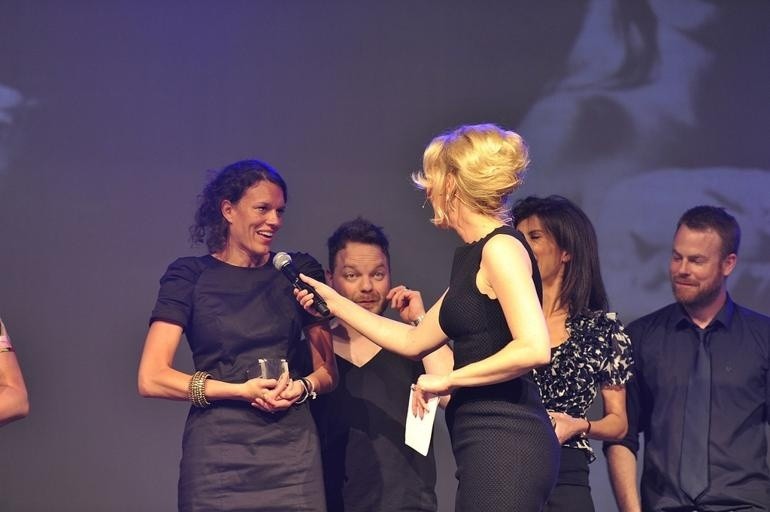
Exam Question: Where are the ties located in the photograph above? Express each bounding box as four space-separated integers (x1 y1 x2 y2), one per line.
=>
679 330 711 501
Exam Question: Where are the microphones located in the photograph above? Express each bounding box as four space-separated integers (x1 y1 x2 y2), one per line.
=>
271 252 331 316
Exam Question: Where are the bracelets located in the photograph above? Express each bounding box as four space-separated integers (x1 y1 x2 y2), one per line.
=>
294 376 314 407
583 418 592 436
188 367 209 410
410 313 426 328
0 337 15 353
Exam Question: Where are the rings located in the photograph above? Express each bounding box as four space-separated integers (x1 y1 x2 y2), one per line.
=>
409 384 417 391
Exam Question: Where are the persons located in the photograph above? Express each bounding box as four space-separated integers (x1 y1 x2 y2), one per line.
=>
603 199 770 511
510 191 635 511
0 314 30 429
295 215 458 511
291 115 562 511
136 155 339 509
